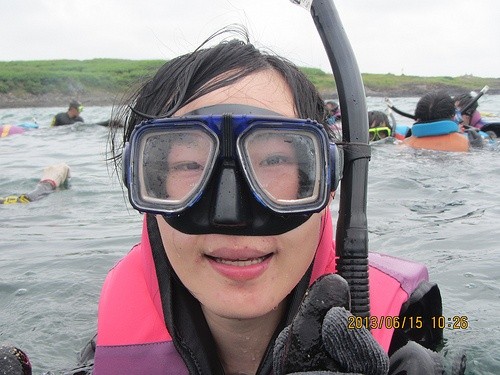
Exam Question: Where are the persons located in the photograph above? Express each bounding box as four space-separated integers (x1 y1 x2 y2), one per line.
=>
451 94 489 136
367 110 412 140
94 23 444 375
403 90 474 151
325 101 343 131
51 99 84 126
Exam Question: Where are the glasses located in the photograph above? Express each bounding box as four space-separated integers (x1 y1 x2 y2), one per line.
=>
369 127 391 142
120 113 342 234
